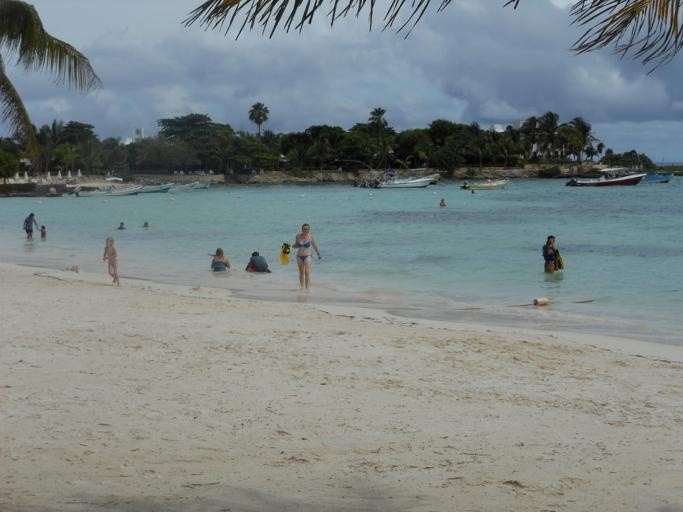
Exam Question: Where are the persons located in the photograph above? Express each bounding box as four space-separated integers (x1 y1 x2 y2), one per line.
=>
36 225 46 239
542 235 563 273
440 198 446 207
143 222 148 227
282 223 322 289
118 222 125 230
103 237 119 286
211 248 231 272
24 213 38 239
245 251 271 273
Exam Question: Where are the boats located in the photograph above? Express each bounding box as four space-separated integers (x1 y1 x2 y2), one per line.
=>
460 177 510 190
568 167 646 187
376 178 432 189
65 180 210 196
418 173 440 184
641 172 675 182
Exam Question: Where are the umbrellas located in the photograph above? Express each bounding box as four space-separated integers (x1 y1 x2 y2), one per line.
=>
14 169 82 182
